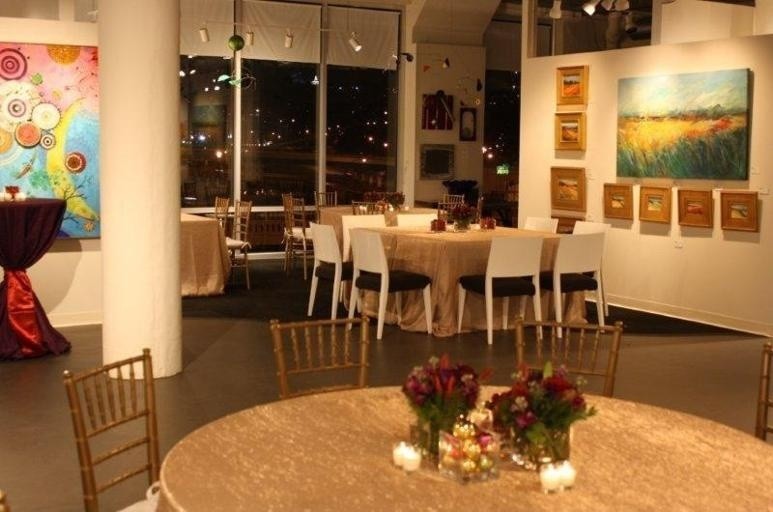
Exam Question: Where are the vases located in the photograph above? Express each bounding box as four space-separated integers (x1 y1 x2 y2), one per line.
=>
524 429 570 466
409 418 452 454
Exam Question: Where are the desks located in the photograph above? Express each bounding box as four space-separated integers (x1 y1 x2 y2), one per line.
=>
0 197 72 364
346 223 586 336
159 382 772 512
179 214 232 299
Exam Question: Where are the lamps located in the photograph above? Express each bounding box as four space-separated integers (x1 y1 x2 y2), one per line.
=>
547 1 637 34
195 18 367 60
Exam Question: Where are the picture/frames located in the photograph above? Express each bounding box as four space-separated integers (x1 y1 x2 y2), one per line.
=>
461 107 477 143
602 182 634 221
554 111 586 151
718 189 758 233
637 185 672 226
555 64 589 107
676 186 714 230
420 144 455 181
550 165 585 218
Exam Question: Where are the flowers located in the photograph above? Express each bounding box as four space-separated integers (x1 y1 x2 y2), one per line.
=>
399 349 480 429
491 369 594 456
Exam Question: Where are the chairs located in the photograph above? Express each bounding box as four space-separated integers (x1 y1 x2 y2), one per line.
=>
511 313 622 397
62 346 161 512
345 227 433 338
540 231 607 338
454 234 547 345
306 222 356 320
267 310 370 398
755 342 773 446
337 211 619 317
211 197 252 287
280 190 486 276
